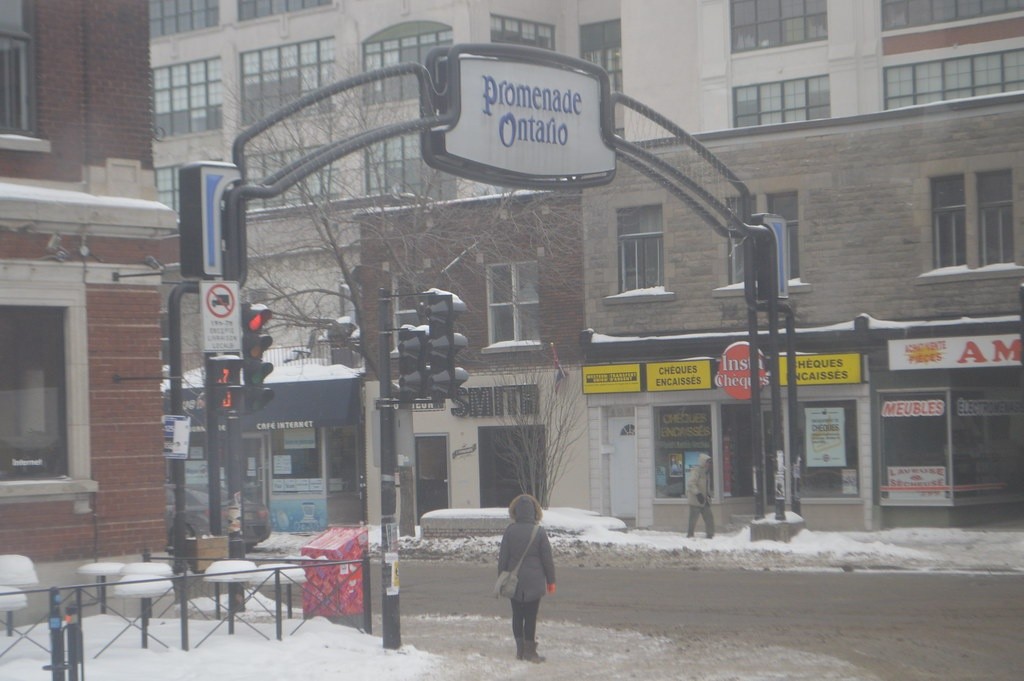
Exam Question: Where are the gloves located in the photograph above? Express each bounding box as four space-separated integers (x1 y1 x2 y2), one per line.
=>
697 493 705 504
547 584 556 594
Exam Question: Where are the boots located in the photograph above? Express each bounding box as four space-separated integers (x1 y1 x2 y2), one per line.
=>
516 638 546 662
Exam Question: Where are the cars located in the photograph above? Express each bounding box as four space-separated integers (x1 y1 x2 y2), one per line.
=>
166 480 271 555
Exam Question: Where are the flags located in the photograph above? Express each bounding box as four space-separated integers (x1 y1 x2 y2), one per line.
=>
551 348 567 394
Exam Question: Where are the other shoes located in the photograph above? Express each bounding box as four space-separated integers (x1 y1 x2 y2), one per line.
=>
707 534 713 539
687 533 693 538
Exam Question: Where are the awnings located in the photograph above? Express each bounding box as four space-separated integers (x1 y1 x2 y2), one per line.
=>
166 373 363 433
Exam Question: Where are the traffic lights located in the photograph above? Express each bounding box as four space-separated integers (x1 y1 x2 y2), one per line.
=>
424 293 470 395
210 357 245 415
243 303 274 412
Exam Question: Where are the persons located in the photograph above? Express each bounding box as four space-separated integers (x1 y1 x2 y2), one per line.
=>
498 494 556 663
686 453 716 539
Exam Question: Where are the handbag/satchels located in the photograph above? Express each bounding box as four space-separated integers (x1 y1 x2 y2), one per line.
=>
494 570 519 599
706 496 713 506
